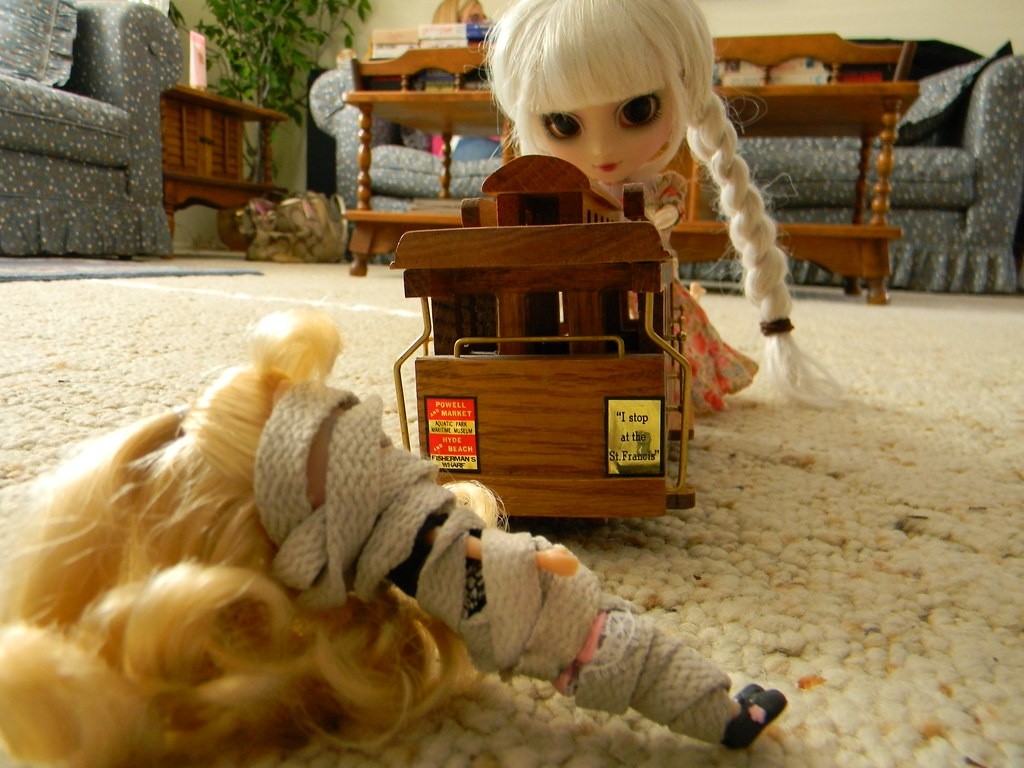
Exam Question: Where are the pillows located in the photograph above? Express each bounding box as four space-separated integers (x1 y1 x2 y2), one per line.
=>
889 39 1013 152
0 0 86 90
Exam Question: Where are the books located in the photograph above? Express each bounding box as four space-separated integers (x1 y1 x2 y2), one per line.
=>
371 22 498 92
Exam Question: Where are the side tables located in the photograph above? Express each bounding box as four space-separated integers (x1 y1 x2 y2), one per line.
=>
156 83 292 261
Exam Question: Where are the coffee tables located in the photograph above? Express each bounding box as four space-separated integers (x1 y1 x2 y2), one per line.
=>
334 30 927 287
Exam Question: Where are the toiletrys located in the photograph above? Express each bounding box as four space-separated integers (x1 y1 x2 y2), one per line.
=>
189 30 207 92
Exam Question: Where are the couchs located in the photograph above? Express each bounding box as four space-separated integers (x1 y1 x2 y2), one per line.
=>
311 38 1024 299
0 0 188 255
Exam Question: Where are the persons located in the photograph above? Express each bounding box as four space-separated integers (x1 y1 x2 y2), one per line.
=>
431 0 505 160
0 315 787 768
489 0 839 420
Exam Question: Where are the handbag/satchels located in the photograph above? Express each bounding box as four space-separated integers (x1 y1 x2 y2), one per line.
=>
240 189 348 263
